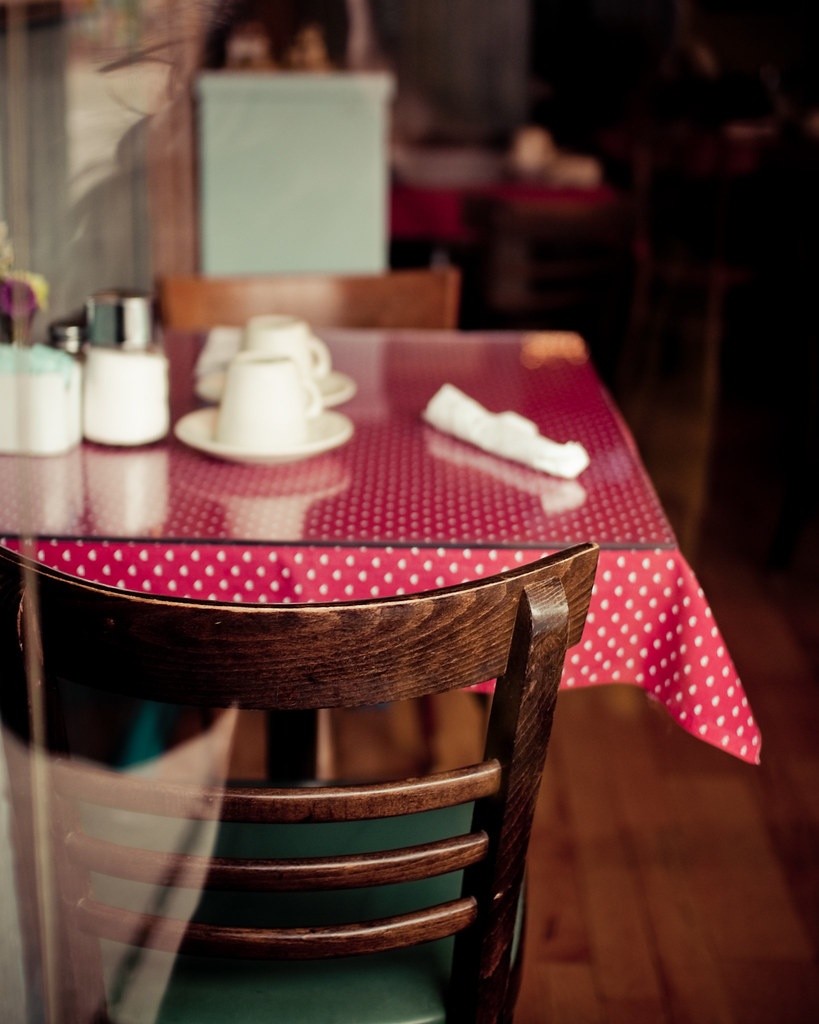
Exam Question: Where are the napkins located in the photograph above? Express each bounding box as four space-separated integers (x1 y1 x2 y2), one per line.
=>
422 382 592 482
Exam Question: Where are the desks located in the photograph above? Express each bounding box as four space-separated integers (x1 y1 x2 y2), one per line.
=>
0 330 765 788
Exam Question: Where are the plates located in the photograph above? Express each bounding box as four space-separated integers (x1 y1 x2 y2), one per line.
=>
174 409 353 462
192 366 356 407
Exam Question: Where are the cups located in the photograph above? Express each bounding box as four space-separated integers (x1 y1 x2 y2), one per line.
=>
84 343 171 446
0 364 85 456
216 348 322 449
246 314 333 387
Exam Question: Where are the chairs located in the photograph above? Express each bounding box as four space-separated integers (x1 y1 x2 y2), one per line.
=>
0 541 600 1024
154 270 463 331
